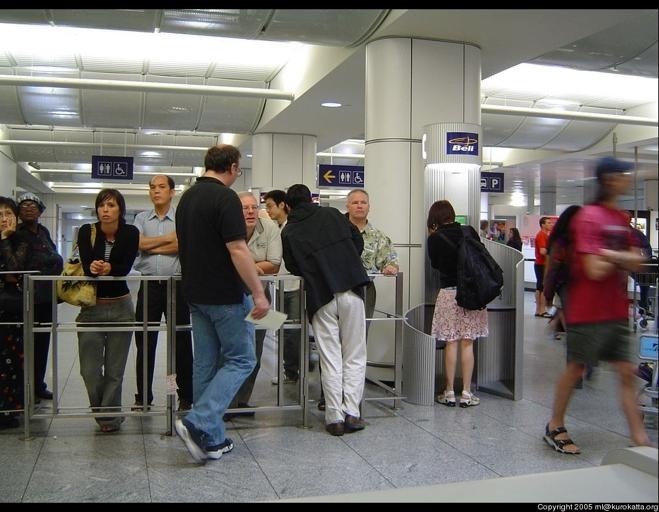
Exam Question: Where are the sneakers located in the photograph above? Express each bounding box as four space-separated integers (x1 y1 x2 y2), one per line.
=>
174 418 209 464
207 438 234 459
271 374 296 384
326 414 366 436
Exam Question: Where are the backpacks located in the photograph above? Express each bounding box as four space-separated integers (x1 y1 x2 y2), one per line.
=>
456 233 504 310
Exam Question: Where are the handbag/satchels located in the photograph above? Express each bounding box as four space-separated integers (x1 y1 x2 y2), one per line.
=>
56 258 98 308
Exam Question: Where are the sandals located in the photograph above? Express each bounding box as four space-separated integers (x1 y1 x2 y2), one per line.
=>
459 390 480 407
438 390 456 407
542 421 581 454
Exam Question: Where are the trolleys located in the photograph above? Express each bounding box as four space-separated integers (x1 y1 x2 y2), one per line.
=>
632 271 658 428
625 255 658 333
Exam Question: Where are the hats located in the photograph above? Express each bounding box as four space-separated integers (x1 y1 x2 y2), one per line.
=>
17 192 46 212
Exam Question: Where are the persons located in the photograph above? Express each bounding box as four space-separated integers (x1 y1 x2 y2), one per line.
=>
16 192 63 404
221 191 283 422
263 190 314 385
280 185 373 436
480 156 657 455
131 174 193 416
173 144 270 465
426 200 489 408
77 188 139 432
317 189 399 411
0 195 34 430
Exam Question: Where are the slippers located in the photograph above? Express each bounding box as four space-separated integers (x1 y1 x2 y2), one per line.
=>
535 312 552 318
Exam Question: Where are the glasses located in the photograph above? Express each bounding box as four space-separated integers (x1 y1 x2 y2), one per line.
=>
21 203 37 209
236 167 242 177
